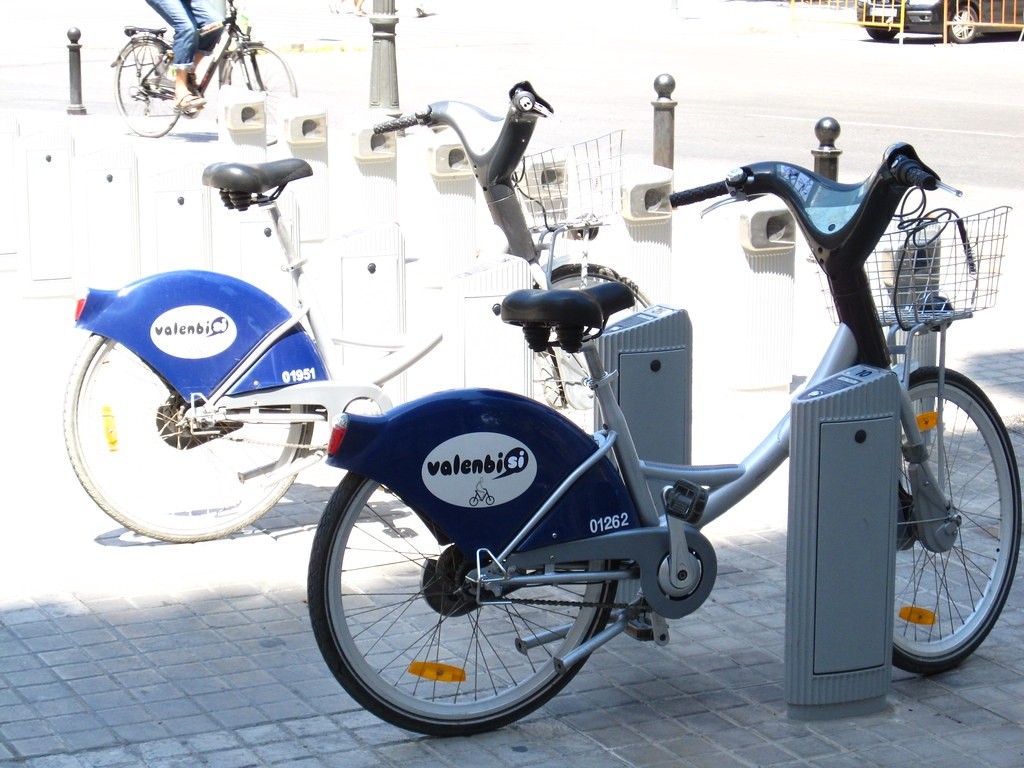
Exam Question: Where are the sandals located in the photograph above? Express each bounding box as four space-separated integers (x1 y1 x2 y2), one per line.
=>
171 93 207 112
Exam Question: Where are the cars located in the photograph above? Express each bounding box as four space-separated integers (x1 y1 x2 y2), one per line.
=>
855 0 1024 43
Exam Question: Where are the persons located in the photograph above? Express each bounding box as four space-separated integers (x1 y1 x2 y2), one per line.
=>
145 0 226 115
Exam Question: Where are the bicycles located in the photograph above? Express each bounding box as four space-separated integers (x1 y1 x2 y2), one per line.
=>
110 0 298 146
307 138 1023 739
57 78 658 545
